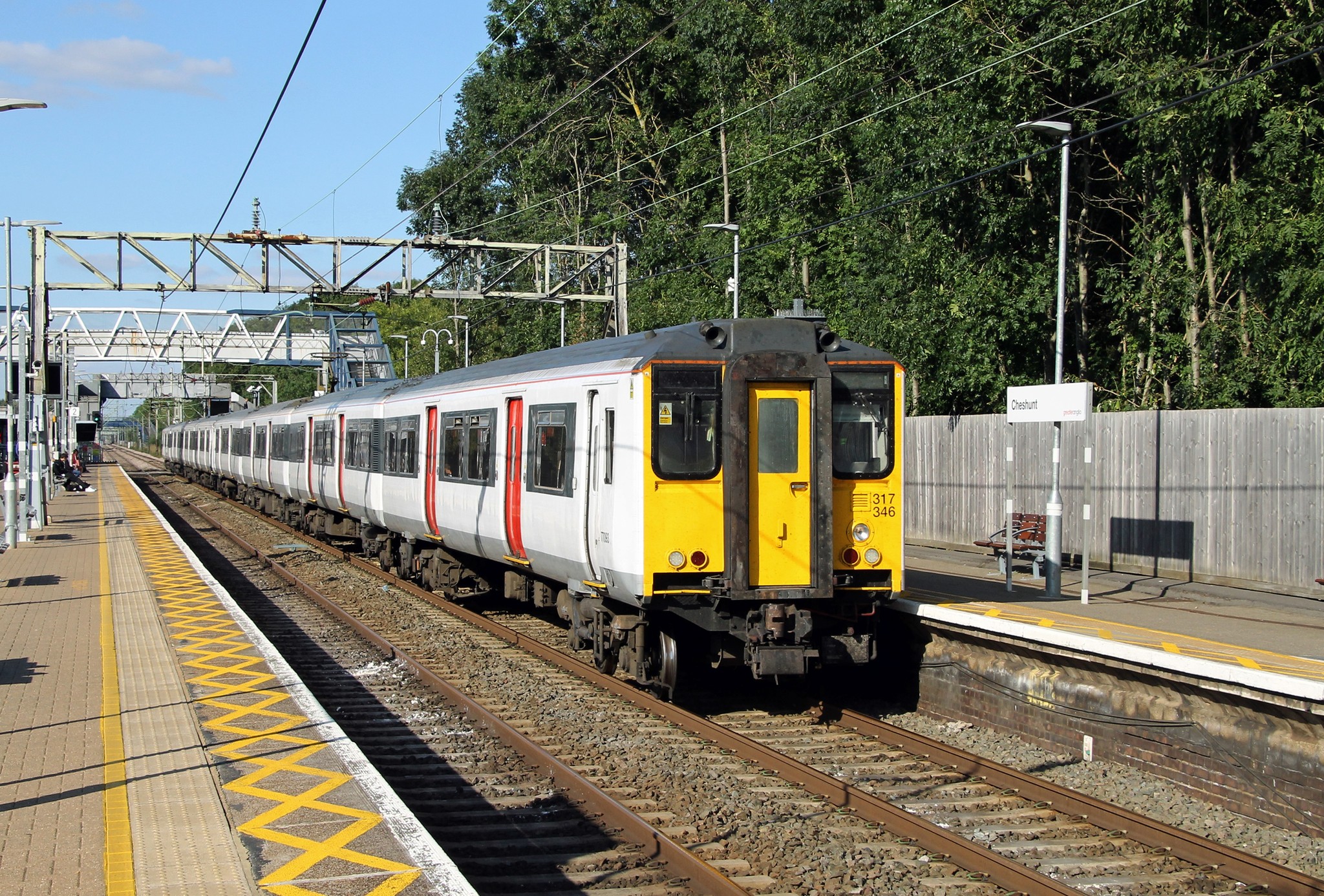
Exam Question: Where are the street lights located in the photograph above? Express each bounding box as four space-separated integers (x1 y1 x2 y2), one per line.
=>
387 335 408 380
1015 119 1071 606
350 347 366 387
702 224 740 320
541 299 565 348
1 216 62 549
264 378 275 404
421 328 454 376
448 315 469 368
313 368 329 393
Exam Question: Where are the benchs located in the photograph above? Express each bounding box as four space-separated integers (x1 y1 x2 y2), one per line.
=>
974 513 1046 580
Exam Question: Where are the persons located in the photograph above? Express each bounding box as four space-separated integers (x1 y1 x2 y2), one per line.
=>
53 453 97 492
445 458 455 477
65 451 81 477
72 449 89 473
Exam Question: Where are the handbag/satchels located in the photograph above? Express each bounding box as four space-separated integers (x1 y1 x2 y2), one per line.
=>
66 484 85 491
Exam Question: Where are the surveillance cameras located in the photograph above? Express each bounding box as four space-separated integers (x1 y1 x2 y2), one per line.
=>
246 386 262 392
32 360 42 369
49 315 54 321
73 362 78 367
421 340 426 346
17 315 23 322
448 340 453 345
68 402 73 406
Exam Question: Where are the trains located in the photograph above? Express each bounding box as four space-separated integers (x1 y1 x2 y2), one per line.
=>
162 319 914 702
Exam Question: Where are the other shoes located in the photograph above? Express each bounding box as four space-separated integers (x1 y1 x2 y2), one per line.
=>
90 485 97 491
85 486 95 492
